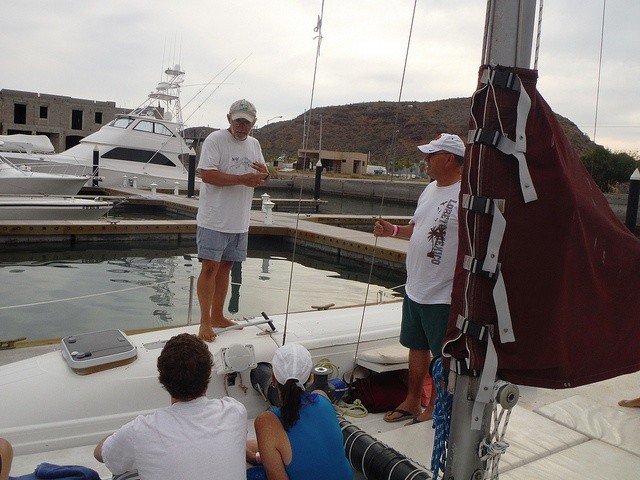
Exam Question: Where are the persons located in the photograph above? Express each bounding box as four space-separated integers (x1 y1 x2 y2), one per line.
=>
94 333 249 480
0 436 15 480
195 99 271 344
373 132 469 427
246 342 354 478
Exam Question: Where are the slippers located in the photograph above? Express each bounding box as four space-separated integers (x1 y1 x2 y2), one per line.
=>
404 416 422 426
384 409 415 422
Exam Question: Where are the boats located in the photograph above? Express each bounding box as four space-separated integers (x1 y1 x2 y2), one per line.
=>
0 154 105 194
0 194 125 220
51 64 202 194
1 0 640 479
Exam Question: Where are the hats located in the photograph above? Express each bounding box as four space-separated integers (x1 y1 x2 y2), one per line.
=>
417 132 466 158
230 99 256 123
272 343 314 391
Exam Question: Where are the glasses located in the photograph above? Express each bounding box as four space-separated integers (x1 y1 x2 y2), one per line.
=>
426 153 452 156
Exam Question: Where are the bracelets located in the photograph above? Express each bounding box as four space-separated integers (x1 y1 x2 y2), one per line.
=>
392 224 398 237
255 452 260 464
